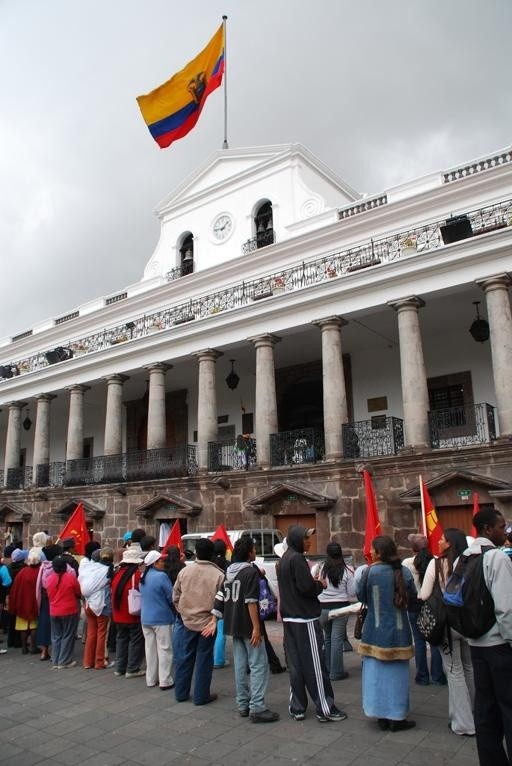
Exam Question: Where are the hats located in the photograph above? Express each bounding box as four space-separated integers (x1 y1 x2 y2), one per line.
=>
274 536 288 558
100 547 114 561
4 544 80 567
184 545 195 554
304 528 316 537
214 538 226 557
60 537 74 547
120 532 168 566
407 533 429 550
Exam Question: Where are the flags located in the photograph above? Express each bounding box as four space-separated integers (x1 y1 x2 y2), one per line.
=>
363 469 384 565
135 23 225 149
418 481 445 557
470 493 480 538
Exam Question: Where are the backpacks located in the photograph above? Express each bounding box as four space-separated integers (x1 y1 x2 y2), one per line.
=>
444 547 497 638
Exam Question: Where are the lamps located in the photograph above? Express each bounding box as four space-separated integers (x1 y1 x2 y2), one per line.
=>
44 347 73 365
225 359 239 392
468 301 489 344
22 408 32 431
0 364 20 378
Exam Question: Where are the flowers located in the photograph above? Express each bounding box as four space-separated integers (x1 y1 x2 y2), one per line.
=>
150 319 165 329
17 361 30 369
69 343 89 350
398 235 418 247
273 277 286 287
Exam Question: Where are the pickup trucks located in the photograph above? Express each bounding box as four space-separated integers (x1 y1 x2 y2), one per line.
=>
181 527 355 614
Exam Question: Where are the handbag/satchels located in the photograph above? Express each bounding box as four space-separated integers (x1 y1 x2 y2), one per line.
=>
354 609 367 639
128 588 141 616
259 579 278 621
415 584 448 648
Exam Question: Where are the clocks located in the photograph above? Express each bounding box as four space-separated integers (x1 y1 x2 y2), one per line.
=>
209 212 235 244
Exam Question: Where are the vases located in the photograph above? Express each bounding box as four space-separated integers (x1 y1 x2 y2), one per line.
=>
272 288 286 297
75 349 86 357
21 368 28 375
402 248 417 257
147 326 159 334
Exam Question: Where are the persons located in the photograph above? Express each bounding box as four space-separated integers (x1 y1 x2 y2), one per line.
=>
242 432 252 469
234 434 249 470
2 505 512 766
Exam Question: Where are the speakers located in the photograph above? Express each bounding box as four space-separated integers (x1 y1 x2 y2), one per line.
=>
439 219 474 244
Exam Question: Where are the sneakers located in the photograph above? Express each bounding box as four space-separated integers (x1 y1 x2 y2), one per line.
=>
208 693 217 704
214 660 230 669
248 709 280 722
0 648 8 654
106 661 115 668
287 705 305 720
125 670 146 678
58 661 76 669
316 710 346 722
273 666 286 674
377 718 415 732
